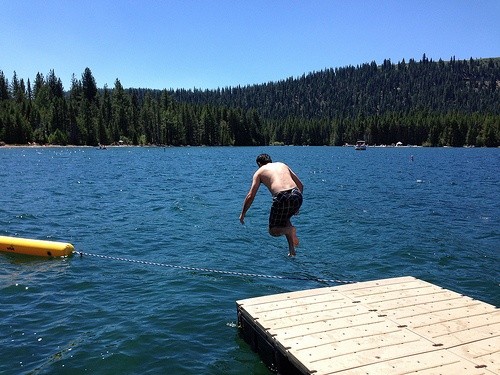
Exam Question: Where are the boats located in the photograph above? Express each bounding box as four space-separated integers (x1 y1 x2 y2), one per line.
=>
355 141 367 150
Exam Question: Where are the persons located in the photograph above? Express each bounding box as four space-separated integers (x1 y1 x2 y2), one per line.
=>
238 152 304 258
